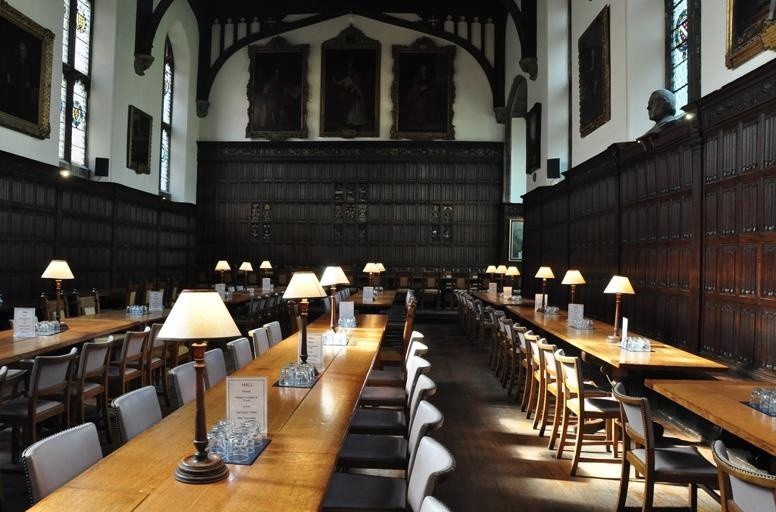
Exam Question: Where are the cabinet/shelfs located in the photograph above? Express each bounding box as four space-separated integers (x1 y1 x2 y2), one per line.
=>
0 149 198 308
699 57 776 388
520 185 564 306
619 103 702 356
561 141 631 328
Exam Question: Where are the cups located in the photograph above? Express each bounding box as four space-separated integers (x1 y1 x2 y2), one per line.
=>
573 318 593 330
319 329 347 345
511 295 522 304
126 304 147 315
224 292 232 301
278 362 316 388
543 306 559 315
206 418 263 461
339 316 357 328
749 385 776 416
622 335 651 352
34 320 61 336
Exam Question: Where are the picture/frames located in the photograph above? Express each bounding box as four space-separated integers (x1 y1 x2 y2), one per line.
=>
577 3 610 139
124 101 154 176
318 21 383 141
524 101 542 176
507 216 524 264
724 0 775 72
244 36 312 143
387 35 458 144
0 1 57 141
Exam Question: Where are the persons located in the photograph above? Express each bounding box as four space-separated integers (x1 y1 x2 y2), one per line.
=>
257 65 297 129
407 62 437 127
5 36 36 122
582 45 600 121
642 85 677 141
329 60 370 129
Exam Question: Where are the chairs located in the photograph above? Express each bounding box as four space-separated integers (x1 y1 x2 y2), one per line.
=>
1 259 776 512
